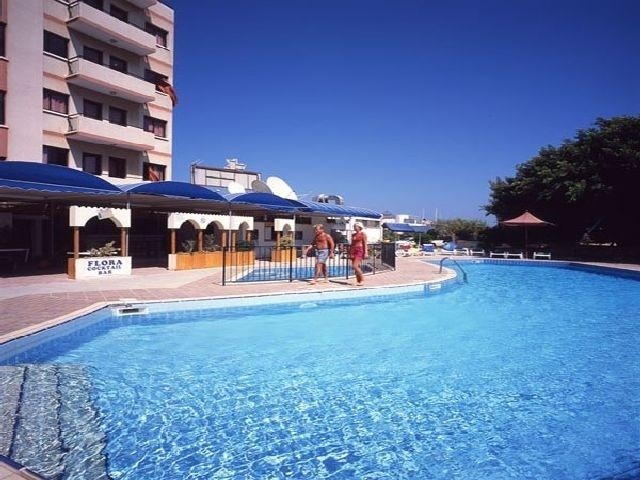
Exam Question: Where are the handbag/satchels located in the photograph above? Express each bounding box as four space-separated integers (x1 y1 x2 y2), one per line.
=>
306 245 319 257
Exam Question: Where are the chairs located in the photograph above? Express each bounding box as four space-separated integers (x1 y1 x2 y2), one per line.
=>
395 243 551 259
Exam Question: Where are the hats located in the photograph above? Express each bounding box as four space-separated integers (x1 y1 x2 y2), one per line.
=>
354 221 364 229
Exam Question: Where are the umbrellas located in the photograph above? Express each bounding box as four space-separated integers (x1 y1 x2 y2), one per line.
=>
500 209 557 258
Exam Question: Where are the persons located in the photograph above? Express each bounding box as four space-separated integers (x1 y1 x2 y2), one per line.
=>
300 223 336 285
348 220 370 287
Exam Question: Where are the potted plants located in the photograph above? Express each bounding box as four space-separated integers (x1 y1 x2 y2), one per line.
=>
168 228 296 270
69 239 133 278
